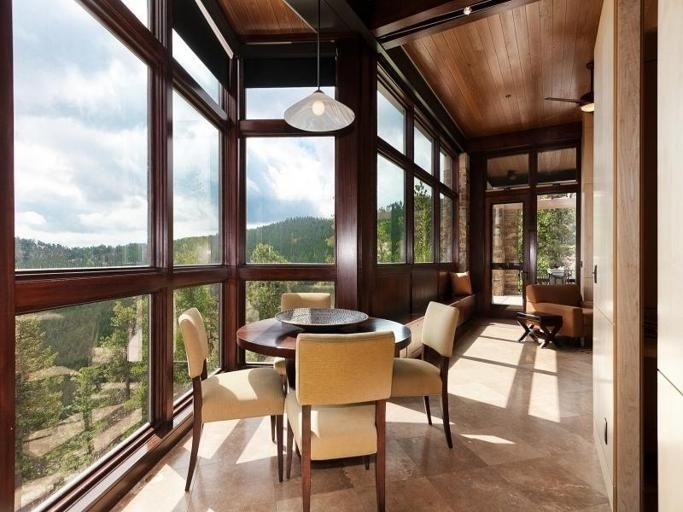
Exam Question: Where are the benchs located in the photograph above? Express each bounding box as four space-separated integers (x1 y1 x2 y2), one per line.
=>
371 292 476 358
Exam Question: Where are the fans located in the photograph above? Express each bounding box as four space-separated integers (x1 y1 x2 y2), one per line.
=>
538 62 593 123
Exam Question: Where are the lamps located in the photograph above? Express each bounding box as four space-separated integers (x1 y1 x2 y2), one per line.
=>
281 1 355 132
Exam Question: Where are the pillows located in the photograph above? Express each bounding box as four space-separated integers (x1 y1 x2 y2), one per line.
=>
449 270 473 296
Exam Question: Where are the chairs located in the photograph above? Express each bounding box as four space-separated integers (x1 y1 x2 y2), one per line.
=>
524 283 593 348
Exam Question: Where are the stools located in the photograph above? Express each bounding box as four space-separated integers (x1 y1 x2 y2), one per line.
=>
512 311 563 349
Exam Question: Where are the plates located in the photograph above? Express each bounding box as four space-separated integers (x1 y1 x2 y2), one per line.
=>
276 307 369 333
